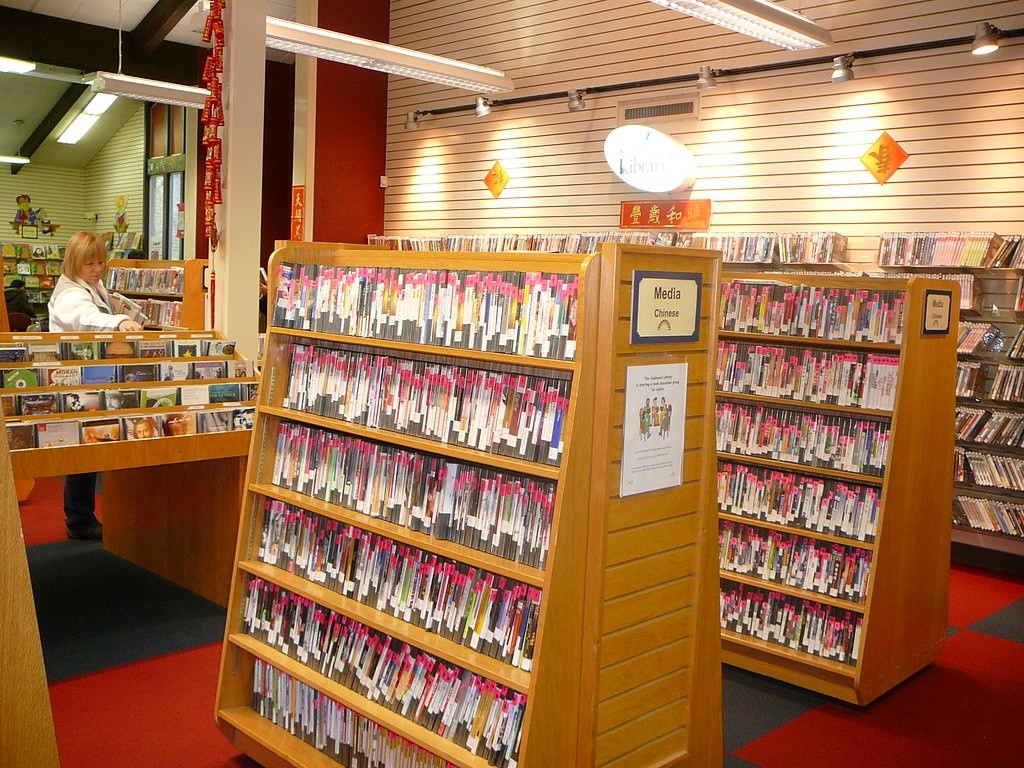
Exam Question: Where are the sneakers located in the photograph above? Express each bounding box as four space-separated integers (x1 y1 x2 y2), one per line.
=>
66 521 103 540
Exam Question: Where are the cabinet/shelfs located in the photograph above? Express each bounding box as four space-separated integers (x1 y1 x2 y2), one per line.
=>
3 242 1024 768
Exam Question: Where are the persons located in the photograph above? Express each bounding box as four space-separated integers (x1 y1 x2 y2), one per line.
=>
94 433 113 441
235 368 241 377
48 231 142 540
70 394 85 412
83 345 92 360
165 366 174 380
259 282 267 315
240 371 246 377
127 249 147 259
135 420 154 438
4 280 37 331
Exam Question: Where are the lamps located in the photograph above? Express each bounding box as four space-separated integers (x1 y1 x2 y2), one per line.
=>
649 0 836 51
404 110 427 129
473 96 496 117
0 56 37 74
604 125 696 193
57 93 118 144
190 4 514 94
832 52 861 81
91 1 211 109
566 91 586 111
695 66 723 89
0 119 30 164
972 22 1004 54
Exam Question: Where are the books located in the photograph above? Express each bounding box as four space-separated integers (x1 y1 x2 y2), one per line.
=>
0 333 265 450
259 268 267 286
2 244 66 303
101 232 141 258
242 232 1024 768
107 267 185 327
